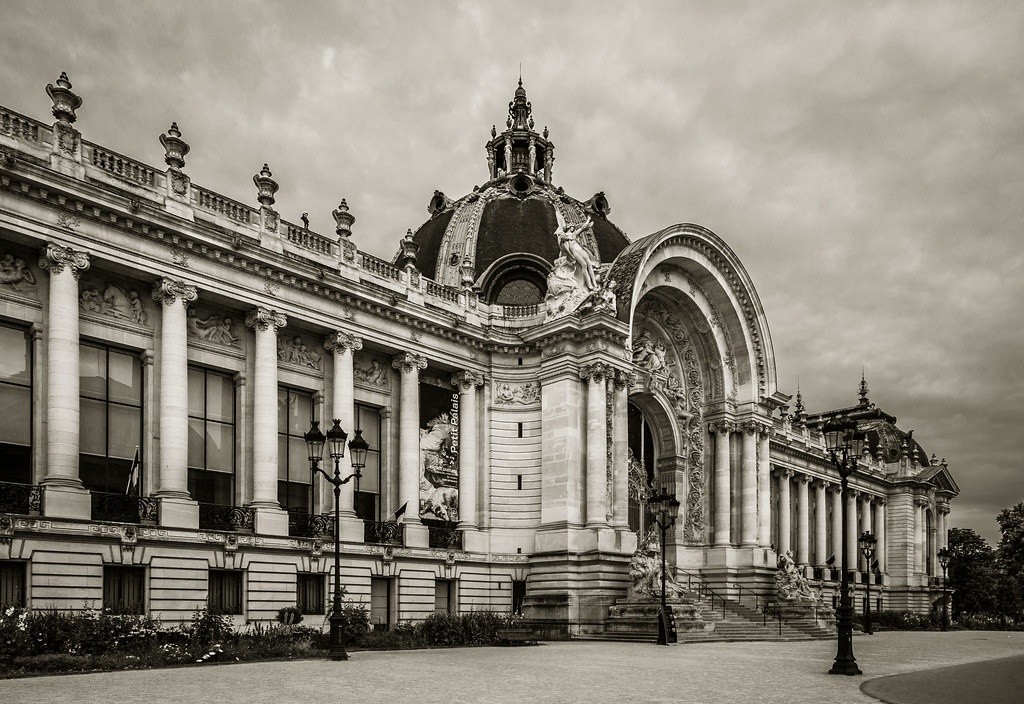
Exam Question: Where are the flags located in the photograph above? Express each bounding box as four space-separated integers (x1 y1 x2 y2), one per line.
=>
131 450 138 490
871 560 878 568
826 555 835 565
395 503 407 526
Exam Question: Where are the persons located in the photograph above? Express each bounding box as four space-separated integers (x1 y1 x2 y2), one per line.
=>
633 331 666 370
301 212 309 229
557 215 598 290
596 281 617 311
774 551 815 595
629 534 672 586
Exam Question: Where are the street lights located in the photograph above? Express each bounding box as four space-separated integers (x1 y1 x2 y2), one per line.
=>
858 529 877 635
820 414 864 674
303 418 370 660
937 546 952 631
647 488 680 643
